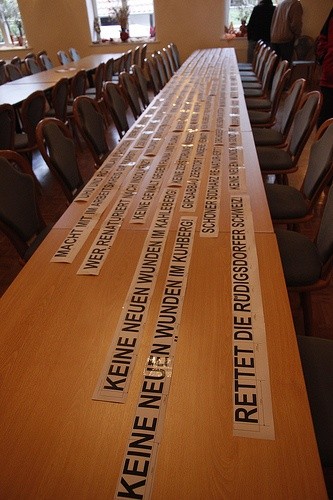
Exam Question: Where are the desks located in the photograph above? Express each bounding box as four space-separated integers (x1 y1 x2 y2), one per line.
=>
0 47 330 500
0 53 124 105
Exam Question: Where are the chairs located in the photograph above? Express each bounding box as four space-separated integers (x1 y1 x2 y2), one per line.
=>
0 34 333 464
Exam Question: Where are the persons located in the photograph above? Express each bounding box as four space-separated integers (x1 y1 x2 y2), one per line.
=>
247 0 275 63
270 0 303 64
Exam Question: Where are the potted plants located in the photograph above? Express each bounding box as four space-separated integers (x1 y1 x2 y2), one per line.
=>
16 20 23 46
113 5 130 42
